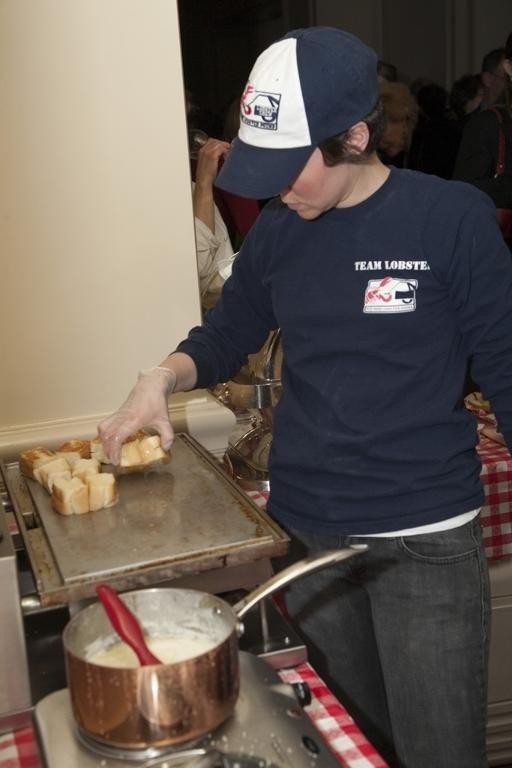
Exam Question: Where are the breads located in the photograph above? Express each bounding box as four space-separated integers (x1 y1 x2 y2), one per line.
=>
19 429 168 515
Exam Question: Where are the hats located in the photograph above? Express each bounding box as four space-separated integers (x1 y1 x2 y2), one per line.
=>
214 25 378 201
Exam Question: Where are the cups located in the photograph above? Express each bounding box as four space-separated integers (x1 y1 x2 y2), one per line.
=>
188 128 208 162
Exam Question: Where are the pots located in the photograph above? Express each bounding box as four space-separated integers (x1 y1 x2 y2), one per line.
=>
62 544 369 749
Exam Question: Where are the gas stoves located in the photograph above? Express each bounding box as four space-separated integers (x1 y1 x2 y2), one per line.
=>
36 651 344 768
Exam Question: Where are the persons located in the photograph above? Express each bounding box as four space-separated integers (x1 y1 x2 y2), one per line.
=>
187 34 512 294
96 24 511 765
